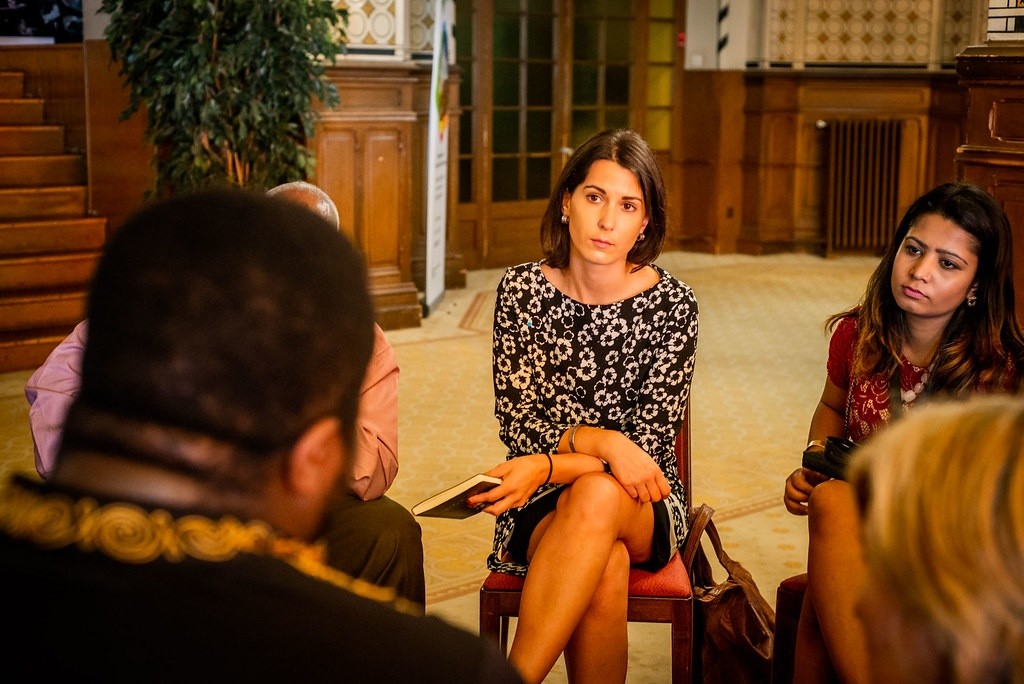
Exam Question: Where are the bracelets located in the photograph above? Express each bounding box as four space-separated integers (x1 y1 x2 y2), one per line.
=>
569 424 587 453
805 440 825 450
540 453 553 487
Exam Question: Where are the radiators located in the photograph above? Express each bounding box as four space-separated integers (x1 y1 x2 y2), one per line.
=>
814 113 906 261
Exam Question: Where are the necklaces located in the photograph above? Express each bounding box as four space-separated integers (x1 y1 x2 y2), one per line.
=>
901 356 938 402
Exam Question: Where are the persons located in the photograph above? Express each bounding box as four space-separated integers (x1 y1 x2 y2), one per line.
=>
0 188 527 684
849 398 1024 684
782 182 1024 684
469 128 699 684
24 183 426 611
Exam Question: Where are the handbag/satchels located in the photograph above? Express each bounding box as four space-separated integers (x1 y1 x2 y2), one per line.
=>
682 502 776 684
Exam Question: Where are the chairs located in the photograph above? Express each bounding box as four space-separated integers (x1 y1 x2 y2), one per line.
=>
482 382 695 684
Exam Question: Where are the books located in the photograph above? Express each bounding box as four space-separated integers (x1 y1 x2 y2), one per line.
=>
411 474 503 520
